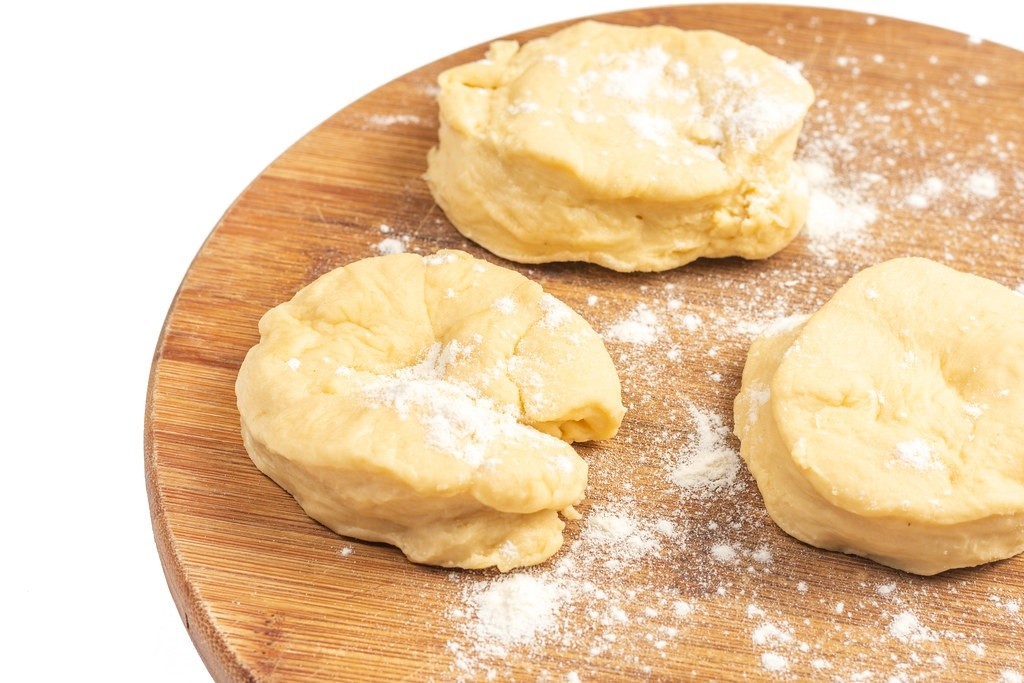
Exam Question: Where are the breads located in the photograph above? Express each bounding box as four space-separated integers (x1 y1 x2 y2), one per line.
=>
419 19 816 274
733 257 1024 576
233 249 622 575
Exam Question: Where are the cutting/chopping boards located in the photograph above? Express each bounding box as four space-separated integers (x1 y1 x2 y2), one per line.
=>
144 2 1024 683
